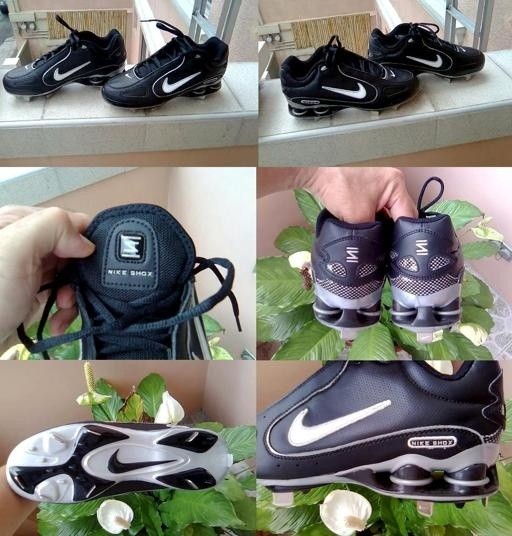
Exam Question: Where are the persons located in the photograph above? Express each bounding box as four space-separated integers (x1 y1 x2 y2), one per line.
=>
257 167 419 229
0 462 49 536
0 202 98 360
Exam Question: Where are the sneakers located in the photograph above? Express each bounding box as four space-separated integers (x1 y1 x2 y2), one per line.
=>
102 19 229 109
2 14 127 98
18 204 240 361
389 176 464 344
280 34 418 118
7 422 234 505
310 208 385 339
256 361 505 503
366 24 485 78
